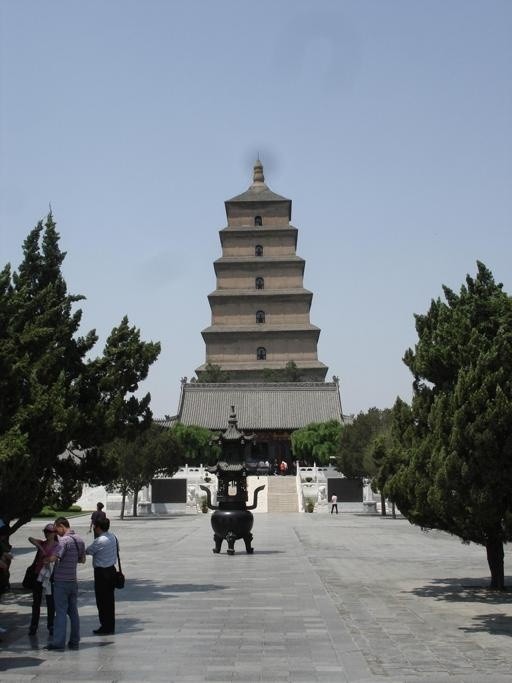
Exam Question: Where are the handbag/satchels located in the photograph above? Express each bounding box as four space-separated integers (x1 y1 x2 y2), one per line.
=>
112 571 125 588
22 550 44 591
68 535 86 564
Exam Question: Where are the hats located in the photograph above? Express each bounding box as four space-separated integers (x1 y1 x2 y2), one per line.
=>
41 523 57 532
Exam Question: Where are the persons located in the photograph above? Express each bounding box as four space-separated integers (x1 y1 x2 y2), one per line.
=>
27 517 86 651
0 517 13 635
89 502 106 539
330 493 338 514
86 518 119 635
258 457 288 476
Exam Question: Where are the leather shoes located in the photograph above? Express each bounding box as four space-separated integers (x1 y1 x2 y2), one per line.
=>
93 626 115 635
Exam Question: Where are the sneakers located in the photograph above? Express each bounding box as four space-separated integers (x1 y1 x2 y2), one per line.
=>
28 627 79 650
